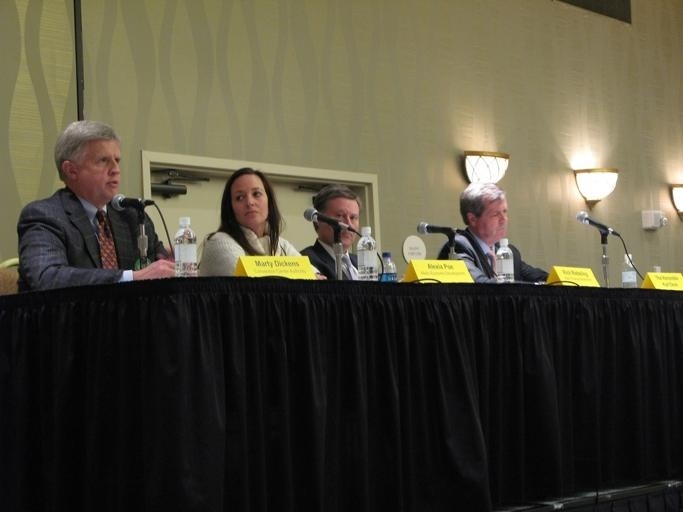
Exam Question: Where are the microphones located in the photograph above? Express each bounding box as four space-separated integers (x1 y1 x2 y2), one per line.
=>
303 207 354 233
576 211 619 237
112 194 157 211
417 220 462 235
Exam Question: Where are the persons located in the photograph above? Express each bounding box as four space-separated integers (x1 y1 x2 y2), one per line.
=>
437 180 551 286
300 181 361 282
16 119 180 294
197 166 328 281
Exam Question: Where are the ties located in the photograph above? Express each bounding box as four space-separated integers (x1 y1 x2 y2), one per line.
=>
94 208 120 269
340 254 359 281
486 250 496 276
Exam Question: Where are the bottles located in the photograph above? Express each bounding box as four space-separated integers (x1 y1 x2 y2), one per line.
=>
378 252 397 282
355 225 379 281
493 237 516 284
173 217 198 277
620 252 637 288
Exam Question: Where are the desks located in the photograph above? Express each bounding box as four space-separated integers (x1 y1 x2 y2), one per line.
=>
0 281 683 507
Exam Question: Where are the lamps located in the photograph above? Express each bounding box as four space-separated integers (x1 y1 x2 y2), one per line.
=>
575 167 618 211
668 183 683 221
465 149 510 185
